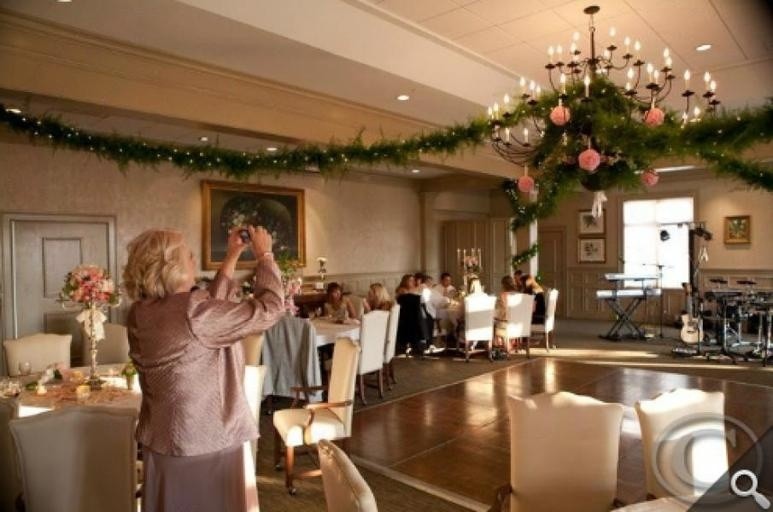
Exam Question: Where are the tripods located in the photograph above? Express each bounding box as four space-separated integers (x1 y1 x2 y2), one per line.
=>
681 298 773 367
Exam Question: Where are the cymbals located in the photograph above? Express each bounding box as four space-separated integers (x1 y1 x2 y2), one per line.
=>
709 278 729 284
736 279 757 285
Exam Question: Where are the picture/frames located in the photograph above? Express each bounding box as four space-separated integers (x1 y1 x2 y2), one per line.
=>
575 208 607 237
575 236 607 265
199 178 308 272
722 215 752 246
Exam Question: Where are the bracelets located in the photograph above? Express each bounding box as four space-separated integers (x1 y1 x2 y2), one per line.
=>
255 252 273 260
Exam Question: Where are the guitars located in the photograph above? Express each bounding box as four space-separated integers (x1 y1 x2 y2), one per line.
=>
681 280 704 346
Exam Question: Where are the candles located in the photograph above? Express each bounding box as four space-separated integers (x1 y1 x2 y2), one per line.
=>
471 247 476 267
477 249 481 268
463 248 466 269
456 247 459 265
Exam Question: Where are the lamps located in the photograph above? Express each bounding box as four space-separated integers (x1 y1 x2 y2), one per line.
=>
656 218 711 241
483 3 725 192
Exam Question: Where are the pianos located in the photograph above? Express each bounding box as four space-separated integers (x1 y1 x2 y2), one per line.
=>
595 272 664 279
592 287 660 299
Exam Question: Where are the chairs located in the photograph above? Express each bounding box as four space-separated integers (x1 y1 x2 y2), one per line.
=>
317 439 378 511
6 402 143 511
633 386 732 501
268 336 361 497
92 322 131 364
250 271 560 416
1 332 73 375
491 389 625 512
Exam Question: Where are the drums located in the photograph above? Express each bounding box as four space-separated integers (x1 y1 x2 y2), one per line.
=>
717 299 740 318
748 311 768 336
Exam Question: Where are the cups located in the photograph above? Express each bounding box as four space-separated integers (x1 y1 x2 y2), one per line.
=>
307 306 321 320
18 361 32 377
0 376 22 399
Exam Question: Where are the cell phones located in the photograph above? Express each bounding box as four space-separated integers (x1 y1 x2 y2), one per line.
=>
239 229 250 243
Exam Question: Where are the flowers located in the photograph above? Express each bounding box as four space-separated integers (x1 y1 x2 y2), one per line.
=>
517 96 666 191
54 265 120 315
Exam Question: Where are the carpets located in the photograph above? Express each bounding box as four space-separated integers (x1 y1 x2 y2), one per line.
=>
256 351 537 511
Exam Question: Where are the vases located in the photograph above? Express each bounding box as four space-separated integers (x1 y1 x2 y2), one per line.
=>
81 312 104 394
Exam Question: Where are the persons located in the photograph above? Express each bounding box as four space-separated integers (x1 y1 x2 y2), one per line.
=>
284 267 549 362
123 225 287 512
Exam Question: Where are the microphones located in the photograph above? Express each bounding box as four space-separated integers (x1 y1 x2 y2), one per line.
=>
619 256 624 264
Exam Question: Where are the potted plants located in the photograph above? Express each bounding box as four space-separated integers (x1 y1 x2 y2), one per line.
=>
121 366 138 389
